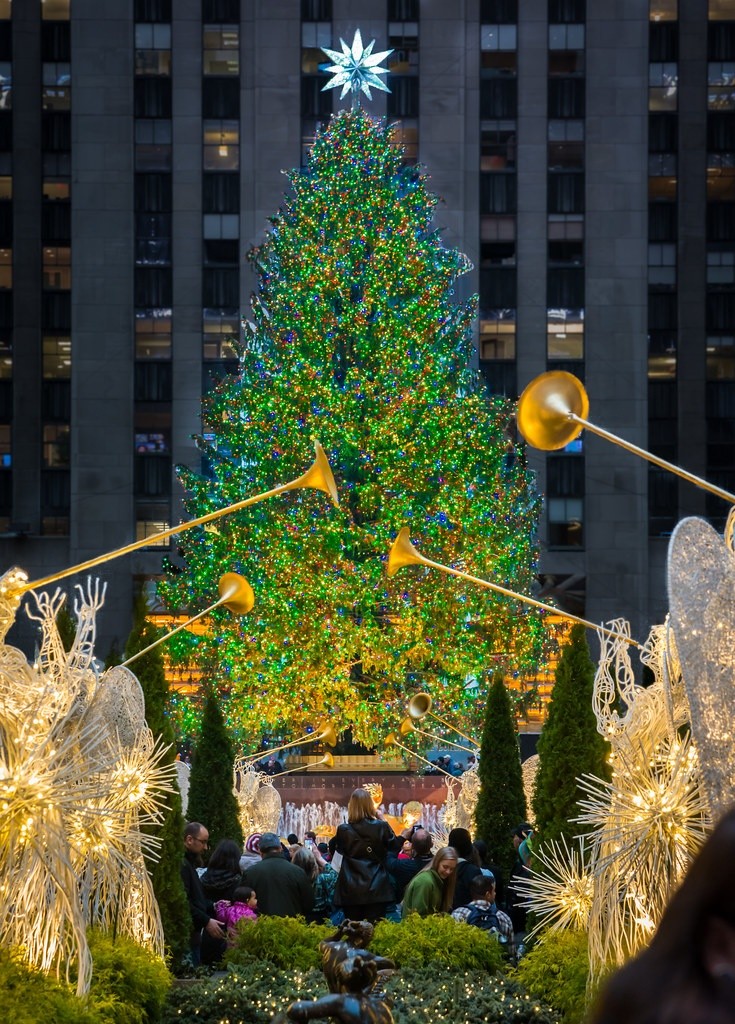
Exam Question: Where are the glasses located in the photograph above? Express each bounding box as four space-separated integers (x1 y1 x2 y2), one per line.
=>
193 837 208 844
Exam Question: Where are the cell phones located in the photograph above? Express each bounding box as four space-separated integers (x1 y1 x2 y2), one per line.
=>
304 839 313 852
413 824 422 833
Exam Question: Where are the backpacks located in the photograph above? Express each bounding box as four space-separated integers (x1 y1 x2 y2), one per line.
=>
460 902 501 935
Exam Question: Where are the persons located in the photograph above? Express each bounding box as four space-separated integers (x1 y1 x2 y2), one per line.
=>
177 789 519 963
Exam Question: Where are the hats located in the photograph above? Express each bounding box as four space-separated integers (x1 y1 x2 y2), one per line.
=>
515 824 533 840
259 832 280 850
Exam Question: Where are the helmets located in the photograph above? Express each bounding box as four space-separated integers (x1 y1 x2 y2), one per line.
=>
246 833 261 855
